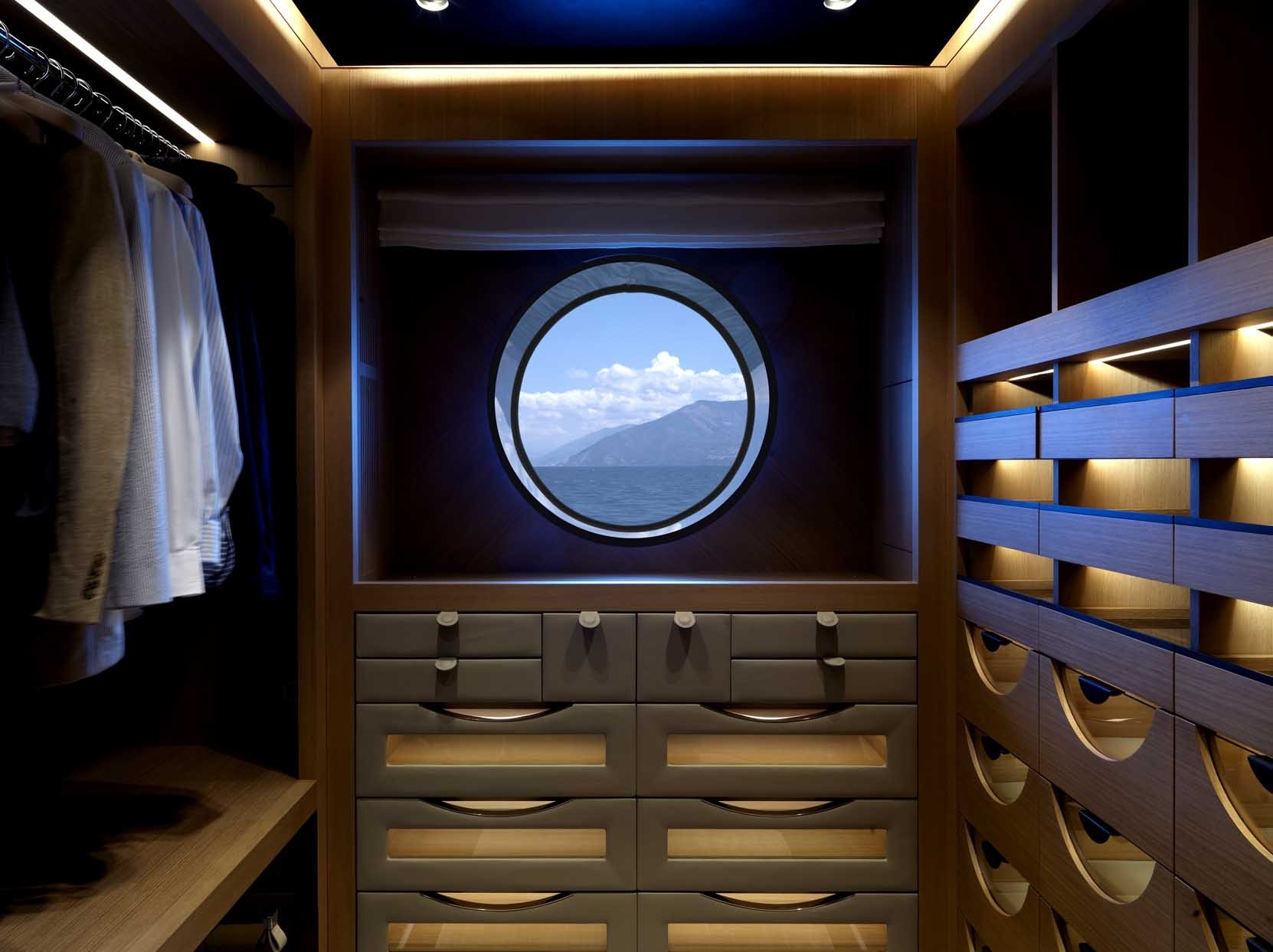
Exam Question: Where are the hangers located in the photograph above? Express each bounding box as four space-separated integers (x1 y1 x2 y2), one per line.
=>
0 20 196 165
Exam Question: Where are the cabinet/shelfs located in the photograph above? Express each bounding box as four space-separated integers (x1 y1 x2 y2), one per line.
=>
945 239 1272 952
353 613 919 952
